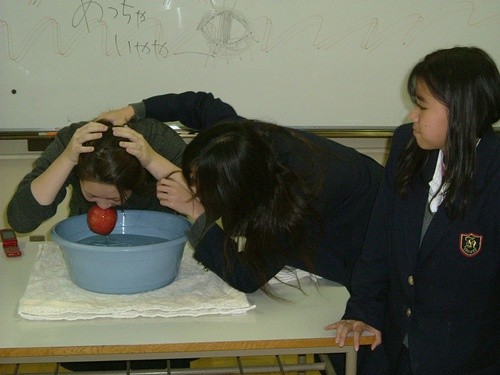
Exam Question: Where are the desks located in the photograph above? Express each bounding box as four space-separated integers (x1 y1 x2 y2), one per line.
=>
0 239 378 375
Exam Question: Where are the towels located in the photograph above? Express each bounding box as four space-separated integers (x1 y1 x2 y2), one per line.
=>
17 240 257 321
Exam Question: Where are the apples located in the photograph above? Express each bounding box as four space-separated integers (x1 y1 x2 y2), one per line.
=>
87 205 117 236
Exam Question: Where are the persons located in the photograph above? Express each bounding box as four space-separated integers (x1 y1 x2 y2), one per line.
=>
7 117 201 372
323 47 500 375
93 91 410 375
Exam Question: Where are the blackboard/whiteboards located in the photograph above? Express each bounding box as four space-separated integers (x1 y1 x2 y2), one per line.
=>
1 0 499 138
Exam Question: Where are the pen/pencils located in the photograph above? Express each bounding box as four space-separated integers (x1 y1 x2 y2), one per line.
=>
38 131 58 136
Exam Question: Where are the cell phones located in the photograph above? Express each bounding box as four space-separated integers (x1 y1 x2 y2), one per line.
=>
0 229 21 257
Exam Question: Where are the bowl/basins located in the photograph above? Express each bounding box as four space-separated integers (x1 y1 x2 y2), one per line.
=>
47 210 192 294
